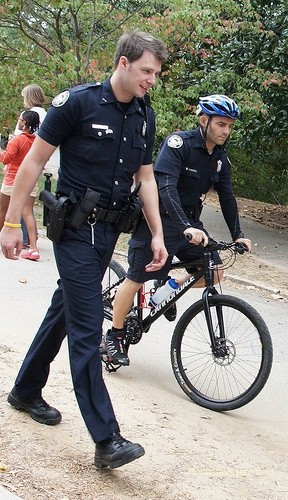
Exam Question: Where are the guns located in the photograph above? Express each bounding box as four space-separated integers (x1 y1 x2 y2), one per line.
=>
39 190 71 242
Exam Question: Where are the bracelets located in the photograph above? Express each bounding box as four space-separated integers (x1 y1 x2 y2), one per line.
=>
4 221 22 228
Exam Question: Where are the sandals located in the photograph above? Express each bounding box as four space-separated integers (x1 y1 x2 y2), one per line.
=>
21 249 39 259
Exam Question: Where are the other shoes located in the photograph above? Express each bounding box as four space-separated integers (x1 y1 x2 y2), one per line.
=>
22 236 38 249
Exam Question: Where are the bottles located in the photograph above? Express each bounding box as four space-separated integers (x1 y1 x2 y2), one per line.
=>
150 278 179 308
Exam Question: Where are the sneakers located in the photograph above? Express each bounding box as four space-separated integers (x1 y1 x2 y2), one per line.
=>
103 329 131 366
154 281 177 321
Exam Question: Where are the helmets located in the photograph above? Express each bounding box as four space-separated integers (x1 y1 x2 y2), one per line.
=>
196 95 239 120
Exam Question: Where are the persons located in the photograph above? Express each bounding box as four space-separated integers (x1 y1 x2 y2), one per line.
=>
0 33 169 469
14 84 60 179
103 94 251 366
0 111 40 259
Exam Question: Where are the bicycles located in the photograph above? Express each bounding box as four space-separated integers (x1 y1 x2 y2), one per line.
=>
98 232 274 412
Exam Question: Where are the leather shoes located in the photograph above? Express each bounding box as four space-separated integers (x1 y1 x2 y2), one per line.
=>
95 432 144 468
7 385 61 425
